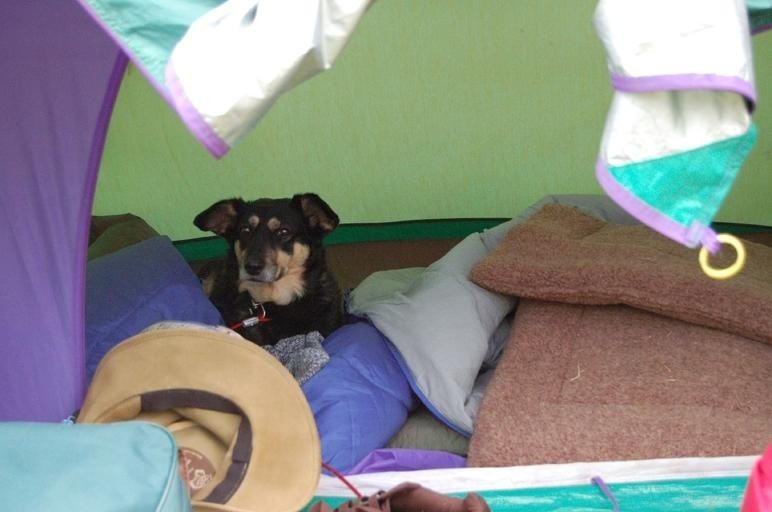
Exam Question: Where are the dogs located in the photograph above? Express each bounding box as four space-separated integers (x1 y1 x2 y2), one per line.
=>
192 192 347 345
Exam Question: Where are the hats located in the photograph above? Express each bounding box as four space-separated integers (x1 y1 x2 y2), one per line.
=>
75 320 323 511
